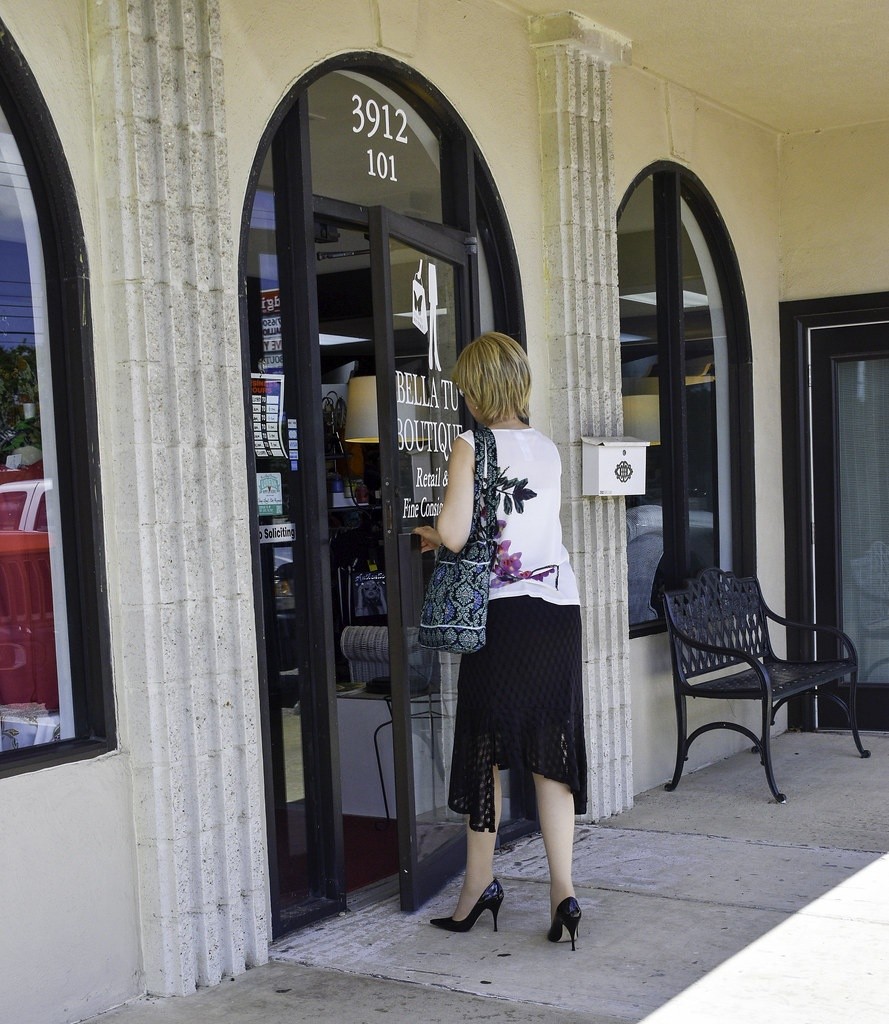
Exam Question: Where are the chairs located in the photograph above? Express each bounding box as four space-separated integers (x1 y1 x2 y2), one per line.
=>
660 567 871 803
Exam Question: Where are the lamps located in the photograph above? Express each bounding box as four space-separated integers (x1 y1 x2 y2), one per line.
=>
341 374 433 443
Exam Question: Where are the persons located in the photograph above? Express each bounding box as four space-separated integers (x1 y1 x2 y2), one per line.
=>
412 332 587 951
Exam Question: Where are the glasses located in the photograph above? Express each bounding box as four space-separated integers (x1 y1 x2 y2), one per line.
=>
458 387 467 397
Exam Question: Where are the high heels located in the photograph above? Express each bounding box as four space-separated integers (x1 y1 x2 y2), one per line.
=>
547 897 581 951
431 875 504 933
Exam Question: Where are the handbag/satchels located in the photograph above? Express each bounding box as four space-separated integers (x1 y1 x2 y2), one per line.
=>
419 426 498 652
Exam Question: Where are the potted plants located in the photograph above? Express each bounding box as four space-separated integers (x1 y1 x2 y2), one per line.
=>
0 423 42 468
